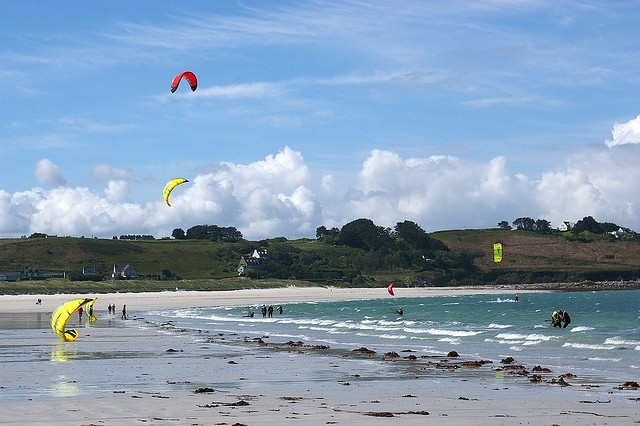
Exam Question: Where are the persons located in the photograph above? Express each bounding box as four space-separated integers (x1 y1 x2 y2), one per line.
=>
559 309 570 328
261 304 267 318
108 305 112 314
268 304 273 318
551 311 562 328
89 305 93 316
112 304 115 314
121 305 127 320
515 295 518 302
278 305 282 314
396 307 403 316
38 297 41 305
243 312 254 317
78 304 83 320
